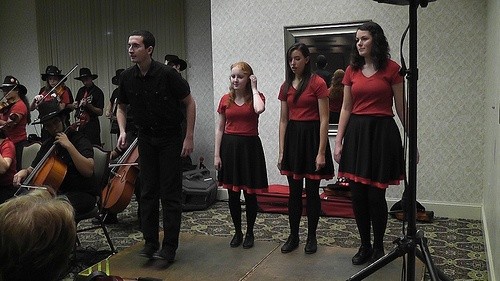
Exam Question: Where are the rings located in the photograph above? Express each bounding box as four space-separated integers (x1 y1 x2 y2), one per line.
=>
253 78 254 79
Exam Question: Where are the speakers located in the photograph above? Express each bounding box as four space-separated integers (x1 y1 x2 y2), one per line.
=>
181 168 218 212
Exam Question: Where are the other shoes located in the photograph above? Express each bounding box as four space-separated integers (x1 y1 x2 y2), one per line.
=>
305 238 317 253
230 232 243 246
243 234 254 248
352 244 373 265
137 243 160 257
92 215 117 224
369 245 385 264
281 237 299 252
153 249 176 260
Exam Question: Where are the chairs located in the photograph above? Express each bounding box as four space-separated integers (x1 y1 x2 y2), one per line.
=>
19 141 42 170
75 145 114 252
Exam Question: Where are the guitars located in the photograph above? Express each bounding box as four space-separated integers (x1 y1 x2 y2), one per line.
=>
75 86 96 119
109 114 118 134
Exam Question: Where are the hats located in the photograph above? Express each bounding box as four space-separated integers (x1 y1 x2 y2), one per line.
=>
73 68 98 80
0 75 27 95
30 100 74 125
112 69 126 85
164 55 187 71
41 66 67 82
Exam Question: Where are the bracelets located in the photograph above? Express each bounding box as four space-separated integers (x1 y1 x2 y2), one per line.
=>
253 92 259 95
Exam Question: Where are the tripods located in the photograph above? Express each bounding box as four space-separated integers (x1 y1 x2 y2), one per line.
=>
344 5 449 281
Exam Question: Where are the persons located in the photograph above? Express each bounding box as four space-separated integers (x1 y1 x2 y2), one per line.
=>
0 186 76 281
328 69 345 124
30 65 74 147
315 55 332 89
13 100 97 219
91 126 139 225
214 61 269 249
277 42 335 254
74 68 104 148
105 69 125 139
164 54 188 75
334 22 419 265
0 128 17 204
0 75 31 171
117 31 197 261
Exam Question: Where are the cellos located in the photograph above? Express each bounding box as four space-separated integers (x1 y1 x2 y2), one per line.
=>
101 138 139 213
14 119 87 198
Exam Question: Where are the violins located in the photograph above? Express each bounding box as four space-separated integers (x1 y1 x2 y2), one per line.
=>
50 84 64 102
0 99 15 117
